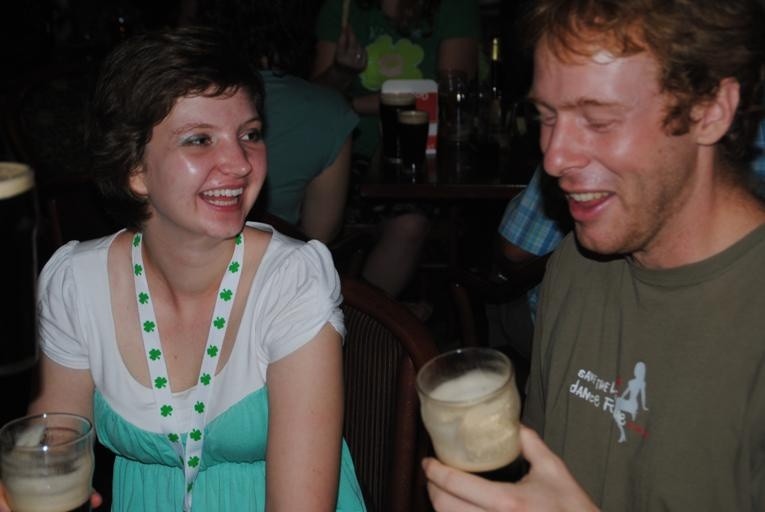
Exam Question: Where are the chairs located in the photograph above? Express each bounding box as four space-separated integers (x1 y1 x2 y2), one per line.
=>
342 279 444 508
465 244 554 372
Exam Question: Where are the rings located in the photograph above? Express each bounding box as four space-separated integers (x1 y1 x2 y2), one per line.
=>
354 52 363 61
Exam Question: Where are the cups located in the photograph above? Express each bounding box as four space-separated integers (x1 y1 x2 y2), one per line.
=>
415 348 531 483
379 91 428 179
0 412 96 512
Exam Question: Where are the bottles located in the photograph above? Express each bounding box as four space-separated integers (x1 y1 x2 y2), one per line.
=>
438 37 512 146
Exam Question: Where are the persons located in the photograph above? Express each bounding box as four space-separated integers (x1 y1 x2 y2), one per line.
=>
306 0 488 120
420 0 765 512
217 2 430 324
484 109 765 390
0 26 368 510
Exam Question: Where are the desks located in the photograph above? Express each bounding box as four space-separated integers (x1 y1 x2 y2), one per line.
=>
349 74 545 198
